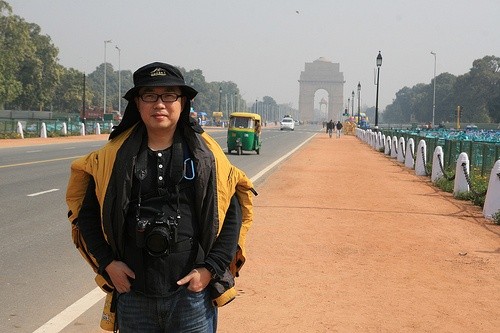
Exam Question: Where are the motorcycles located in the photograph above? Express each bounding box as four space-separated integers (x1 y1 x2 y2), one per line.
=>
226 112 262 155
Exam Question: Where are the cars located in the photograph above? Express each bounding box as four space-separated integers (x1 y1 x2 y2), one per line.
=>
294 119 299 126
279 117 295 131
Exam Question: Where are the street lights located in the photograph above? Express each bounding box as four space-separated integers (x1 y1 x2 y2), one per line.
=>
219 87 222 111
234 91 237 112
347 97 350 114
103 40 113 114
430 51 437 128
351 89 354 117
115 45 121 114
374 50 383 130
357 81 361 127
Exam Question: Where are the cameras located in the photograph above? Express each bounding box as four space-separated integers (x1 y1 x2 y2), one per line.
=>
136 212 178 257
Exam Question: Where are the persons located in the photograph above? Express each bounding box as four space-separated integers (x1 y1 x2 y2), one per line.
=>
421 121 449 130
299 120 343 138
77 62 242 333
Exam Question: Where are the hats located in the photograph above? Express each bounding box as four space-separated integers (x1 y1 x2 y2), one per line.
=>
122 62 199 102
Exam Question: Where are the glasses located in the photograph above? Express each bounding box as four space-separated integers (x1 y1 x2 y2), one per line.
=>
137 92 182 102
